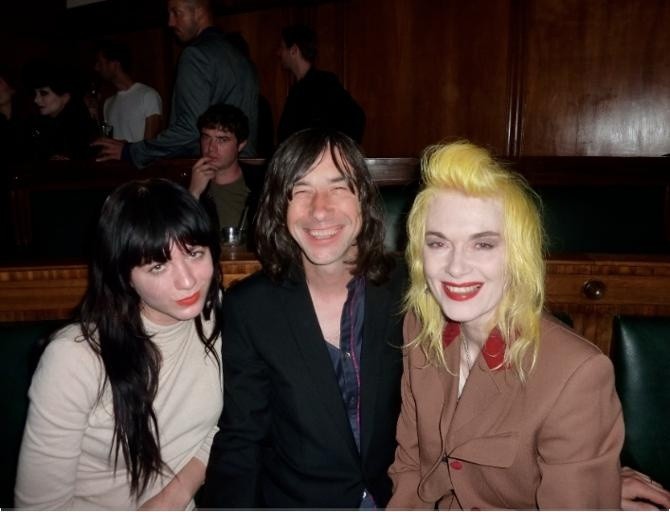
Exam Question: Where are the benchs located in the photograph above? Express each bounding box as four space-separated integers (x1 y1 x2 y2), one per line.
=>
1 155 670 511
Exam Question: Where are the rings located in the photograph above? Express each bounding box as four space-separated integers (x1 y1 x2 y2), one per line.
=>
649 478 654 485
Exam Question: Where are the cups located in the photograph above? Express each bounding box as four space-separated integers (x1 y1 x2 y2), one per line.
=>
95 125 113 141
221 225 247 257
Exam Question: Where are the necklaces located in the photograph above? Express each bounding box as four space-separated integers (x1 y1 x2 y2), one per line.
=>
460 334 473 375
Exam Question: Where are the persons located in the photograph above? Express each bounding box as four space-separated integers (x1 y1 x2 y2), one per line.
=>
26 57 92 170
1 62 26 175
11 173 229 512
86 1 263 171
268 23 368 152
183 102 266 247
382 136 624 510
93 38 165 144
194 120 668 511
